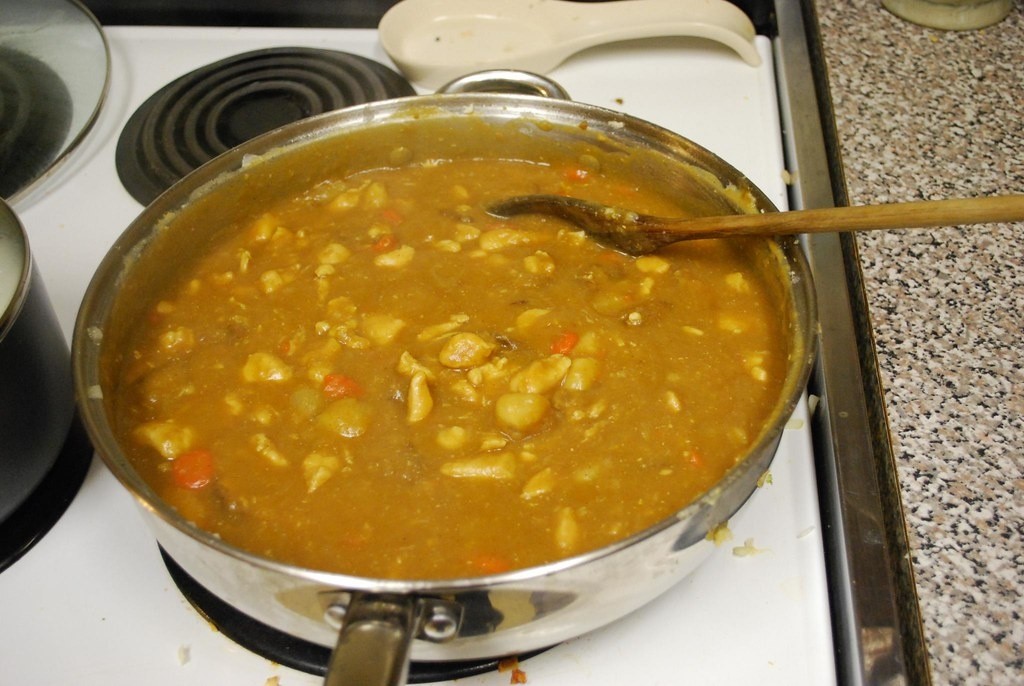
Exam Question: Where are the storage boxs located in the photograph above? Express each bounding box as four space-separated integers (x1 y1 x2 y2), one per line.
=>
70 68 817 685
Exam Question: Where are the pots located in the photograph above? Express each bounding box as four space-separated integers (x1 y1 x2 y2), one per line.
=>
0 195 77 522
74 60 823 685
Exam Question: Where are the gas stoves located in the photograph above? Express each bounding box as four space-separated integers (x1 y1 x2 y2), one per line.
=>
3 1 913 685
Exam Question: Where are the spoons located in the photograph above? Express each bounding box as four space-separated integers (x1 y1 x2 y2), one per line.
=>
496 181 1018 252
377 0 752 90
